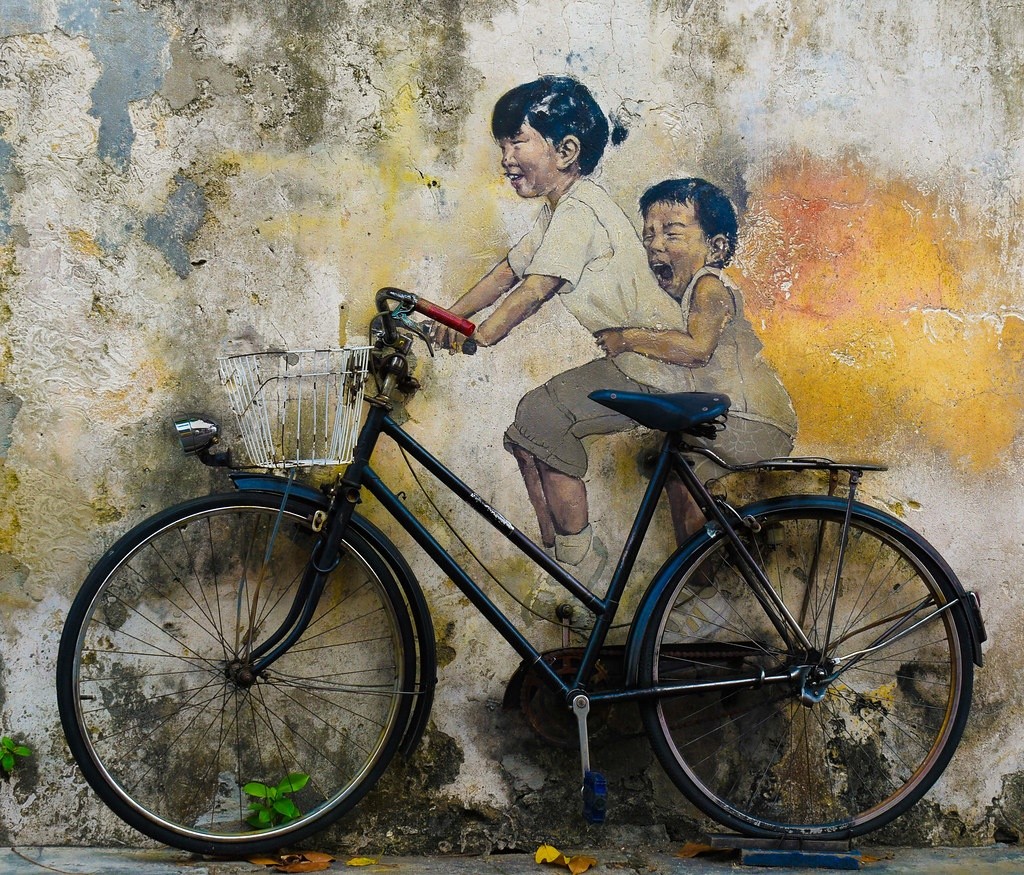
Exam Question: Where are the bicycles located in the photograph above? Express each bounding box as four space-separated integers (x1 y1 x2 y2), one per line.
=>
58 284 990 863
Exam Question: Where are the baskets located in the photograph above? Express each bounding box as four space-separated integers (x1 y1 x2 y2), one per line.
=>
215 344 377 468
